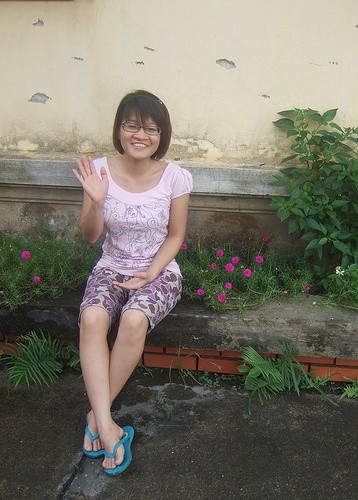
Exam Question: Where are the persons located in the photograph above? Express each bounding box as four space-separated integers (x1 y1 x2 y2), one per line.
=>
71 90 194 476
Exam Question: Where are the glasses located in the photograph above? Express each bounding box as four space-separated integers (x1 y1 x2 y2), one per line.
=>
120 120 164 135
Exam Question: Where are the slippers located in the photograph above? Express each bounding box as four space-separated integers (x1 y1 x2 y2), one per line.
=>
81 415 105 459
103 426 134 475
